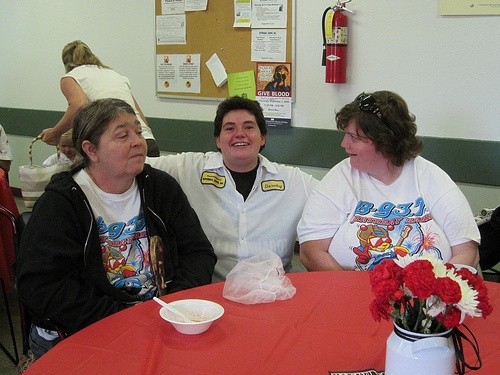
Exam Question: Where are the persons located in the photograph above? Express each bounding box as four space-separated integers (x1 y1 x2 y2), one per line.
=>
0 125 12 183
143 96 320 282
297 91 481 271
42 129 83 165
38 40 159 157
16 99 217 360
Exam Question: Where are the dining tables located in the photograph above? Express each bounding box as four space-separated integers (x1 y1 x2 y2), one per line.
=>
20 270 500 375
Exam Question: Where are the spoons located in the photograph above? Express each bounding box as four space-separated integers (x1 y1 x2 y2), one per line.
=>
153 296 194 322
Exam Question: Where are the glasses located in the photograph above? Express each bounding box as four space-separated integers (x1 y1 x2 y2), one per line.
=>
354 92 389 128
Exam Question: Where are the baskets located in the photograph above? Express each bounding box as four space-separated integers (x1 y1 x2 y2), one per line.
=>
19 137 71 208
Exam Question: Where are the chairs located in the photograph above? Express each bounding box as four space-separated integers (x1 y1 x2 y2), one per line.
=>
0 205 22 366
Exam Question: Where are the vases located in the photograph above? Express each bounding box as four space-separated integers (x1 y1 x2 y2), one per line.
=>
384 319 458 375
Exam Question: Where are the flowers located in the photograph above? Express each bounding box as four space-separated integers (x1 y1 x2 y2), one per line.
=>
368 253 493 330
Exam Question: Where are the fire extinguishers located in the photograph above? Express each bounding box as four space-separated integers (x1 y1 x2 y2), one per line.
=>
321 0 354 84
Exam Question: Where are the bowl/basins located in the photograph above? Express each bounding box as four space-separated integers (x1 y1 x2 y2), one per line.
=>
159 298 224 334
